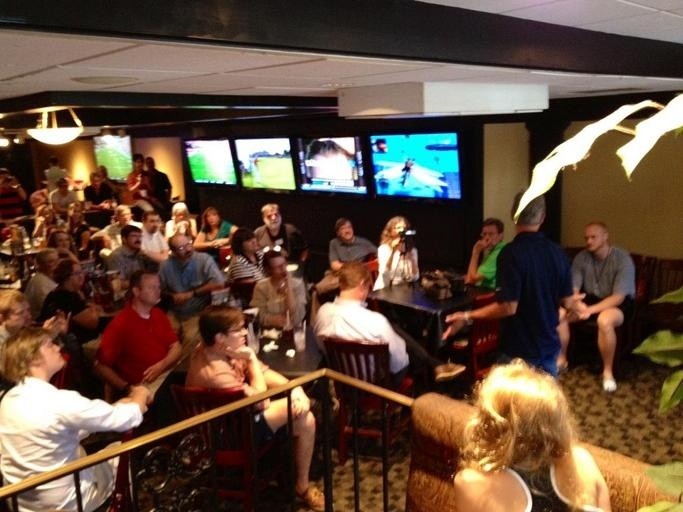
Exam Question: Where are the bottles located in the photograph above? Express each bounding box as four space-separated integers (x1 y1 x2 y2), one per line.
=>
279 306 296 357
246 321 259 354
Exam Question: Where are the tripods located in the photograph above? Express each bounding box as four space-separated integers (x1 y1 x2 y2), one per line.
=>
389 251 415 289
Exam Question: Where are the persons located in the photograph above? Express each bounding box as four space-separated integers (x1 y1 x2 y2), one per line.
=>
554 219 637 394
0 153 510 452
452 359 614 511
182 300 328 510
436 185 587 377
2 319 151 511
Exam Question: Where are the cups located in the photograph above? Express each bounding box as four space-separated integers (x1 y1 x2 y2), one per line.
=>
292 320 306 351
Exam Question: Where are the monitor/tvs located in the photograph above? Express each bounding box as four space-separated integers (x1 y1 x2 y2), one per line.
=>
294 134 368 194
182 139 236 185
370 133 462 200
92 134 134 183
235 137 295 191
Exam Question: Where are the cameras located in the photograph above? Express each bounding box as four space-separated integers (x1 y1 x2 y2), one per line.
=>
400 228 421 251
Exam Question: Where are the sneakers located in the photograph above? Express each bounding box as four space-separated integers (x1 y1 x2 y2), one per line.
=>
435 363 466 381
295 482 325 511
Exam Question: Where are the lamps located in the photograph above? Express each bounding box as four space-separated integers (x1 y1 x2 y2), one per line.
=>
27 107 84 145
